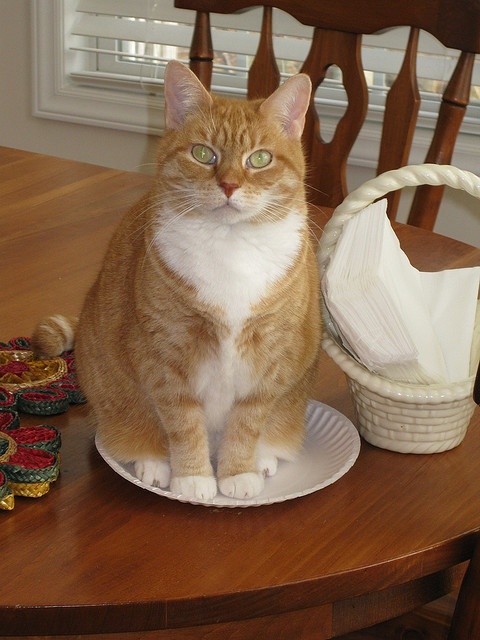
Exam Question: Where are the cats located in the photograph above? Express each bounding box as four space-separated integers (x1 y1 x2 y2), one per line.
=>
31 60 325 500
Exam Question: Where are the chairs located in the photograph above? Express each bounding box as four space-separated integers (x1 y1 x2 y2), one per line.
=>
174 0 480 233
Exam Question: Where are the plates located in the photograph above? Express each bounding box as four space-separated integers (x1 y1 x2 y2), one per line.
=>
93 393 362 505
